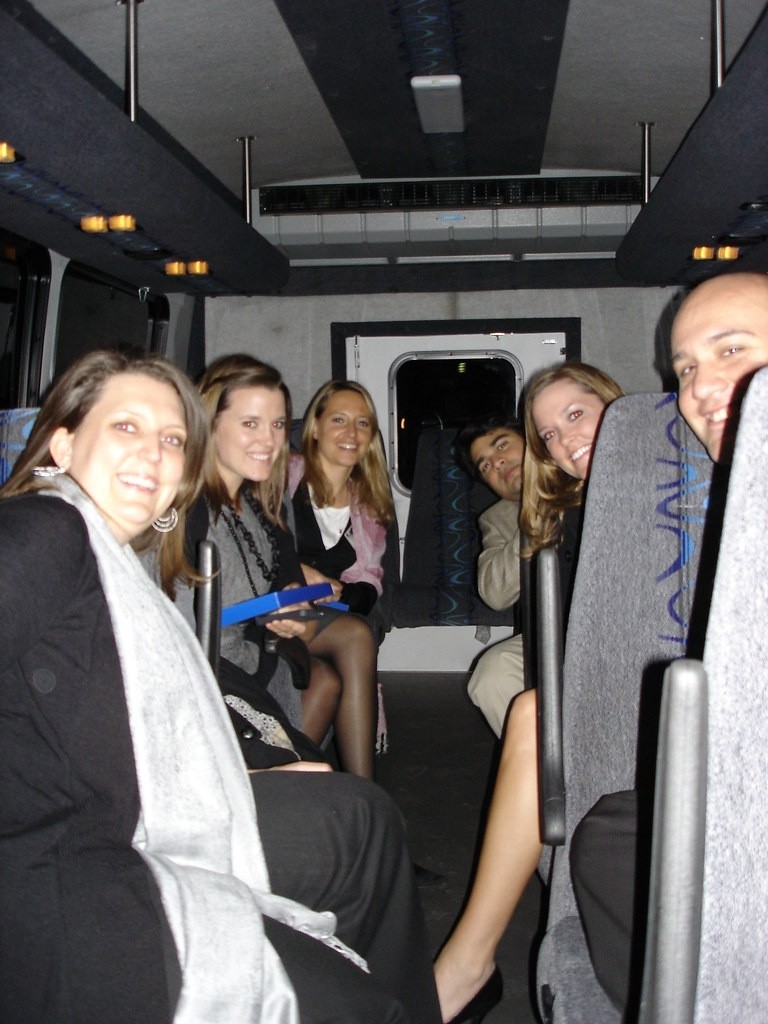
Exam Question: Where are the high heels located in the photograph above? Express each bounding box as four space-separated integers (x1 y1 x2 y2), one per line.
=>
448 963 505 1024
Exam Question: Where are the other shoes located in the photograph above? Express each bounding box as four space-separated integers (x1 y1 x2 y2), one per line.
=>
414 863 449 890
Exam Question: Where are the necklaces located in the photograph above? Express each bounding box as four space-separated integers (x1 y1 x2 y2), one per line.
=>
217 491 279 597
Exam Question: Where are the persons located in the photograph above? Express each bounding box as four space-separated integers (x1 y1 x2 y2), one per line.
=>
431 361 627 1024
288 380 401 782
0 346 442 1024
568 257 768 1024
455 416 527 741
133 355 332 773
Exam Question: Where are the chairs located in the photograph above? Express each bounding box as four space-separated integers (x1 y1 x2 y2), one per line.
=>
533 394 722 1024
0 406 225 677
639 362 768 1024
